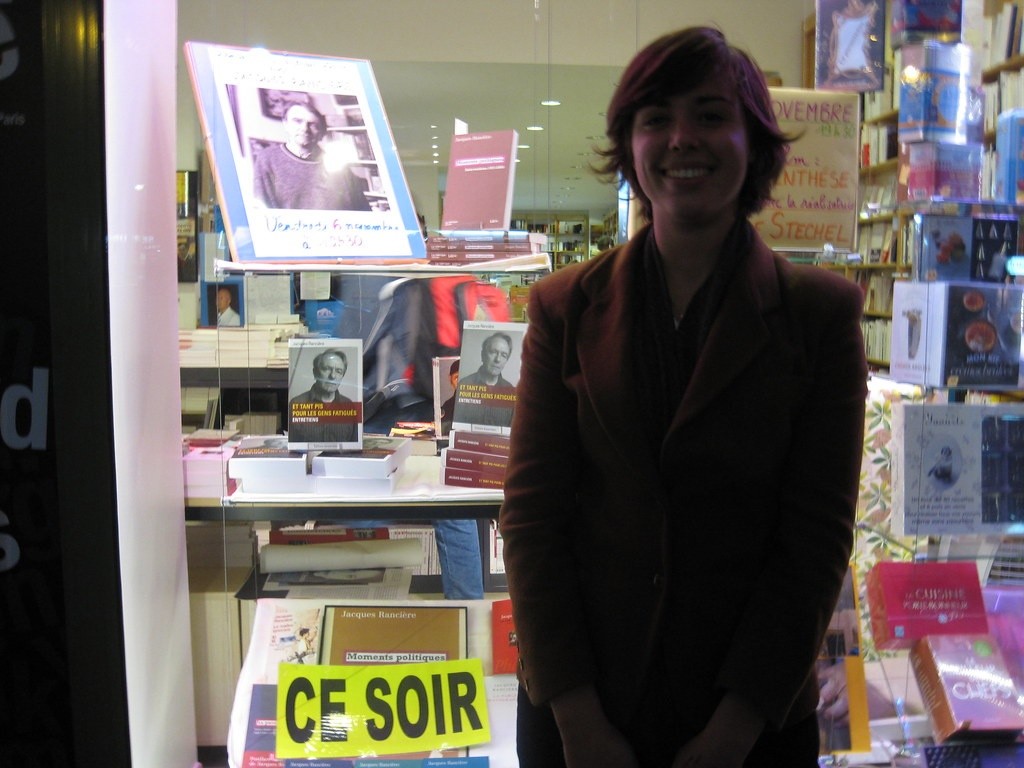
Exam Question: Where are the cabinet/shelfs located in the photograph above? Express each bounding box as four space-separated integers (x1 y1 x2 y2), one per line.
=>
175 0 1024 768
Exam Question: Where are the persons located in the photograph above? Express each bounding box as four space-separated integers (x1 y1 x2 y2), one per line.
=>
498 25 869 768
335 273 485 601
253 89 368 209
290 350 359 443
214 287 238 326
442 360 462 436
452 332 517 425
177 235 196 281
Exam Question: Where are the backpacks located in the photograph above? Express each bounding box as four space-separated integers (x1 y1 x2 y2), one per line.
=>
362 273 513 430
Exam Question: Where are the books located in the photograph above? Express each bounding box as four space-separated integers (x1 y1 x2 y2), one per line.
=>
177 151 309 367
287 338 363 451
188 523 521 768
181 412 414 499
429 230 548 269
184 41 426 265
387 320 529 488
815 0 1024 768
438 129 519 230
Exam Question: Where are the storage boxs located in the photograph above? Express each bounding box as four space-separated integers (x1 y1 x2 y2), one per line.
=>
229 436 309 480
314 461 406 496
311 436 413 476
889 282 1023 390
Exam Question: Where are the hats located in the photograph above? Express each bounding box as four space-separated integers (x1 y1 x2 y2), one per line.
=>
450 360 459 374
